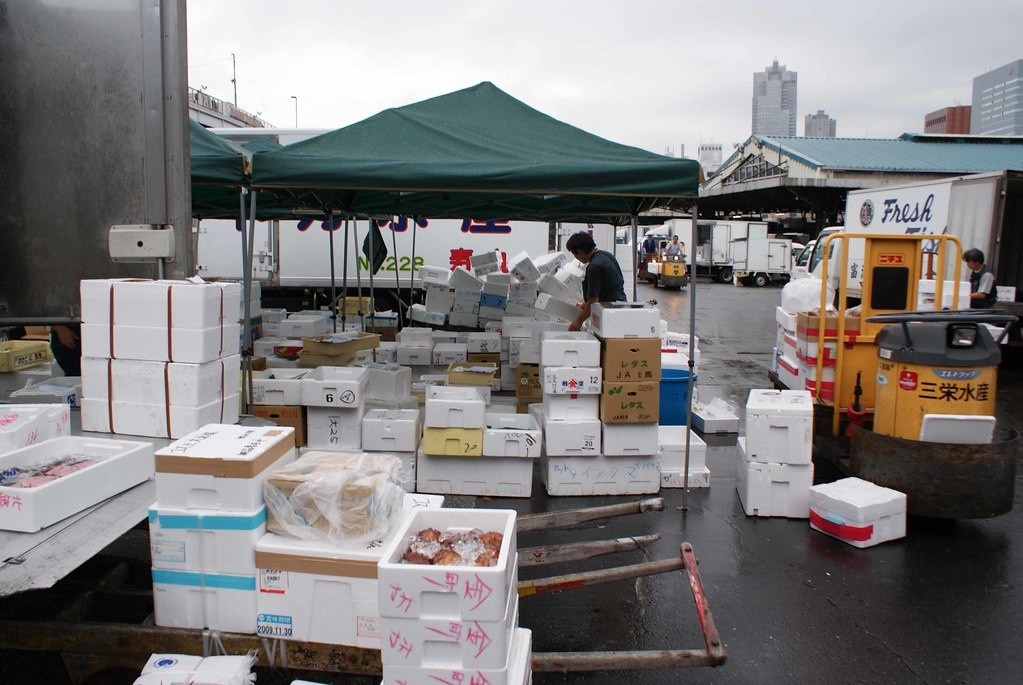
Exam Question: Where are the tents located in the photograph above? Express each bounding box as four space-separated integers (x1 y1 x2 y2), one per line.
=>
187 81 701 509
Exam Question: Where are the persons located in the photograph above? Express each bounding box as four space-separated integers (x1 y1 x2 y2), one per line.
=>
962 247 998 310
50 326 82 377
665 235 683 261
642 235 658 254
566 231 628 332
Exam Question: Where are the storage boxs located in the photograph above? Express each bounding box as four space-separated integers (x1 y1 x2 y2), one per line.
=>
0 250 1017 685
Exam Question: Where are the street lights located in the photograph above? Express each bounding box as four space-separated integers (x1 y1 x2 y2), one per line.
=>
290 96 298 129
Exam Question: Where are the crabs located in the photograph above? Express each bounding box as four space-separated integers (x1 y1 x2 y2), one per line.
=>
400 527 503 567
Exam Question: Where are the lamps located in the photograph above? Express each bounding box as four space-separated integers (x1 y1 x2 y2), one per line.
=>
732 143 741 152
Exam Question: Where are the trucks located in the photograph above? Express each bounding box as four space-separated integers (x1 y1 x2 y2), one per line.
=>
1 1 814 336
789 168 1023 379
